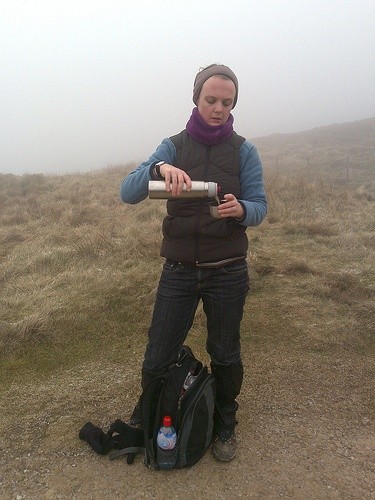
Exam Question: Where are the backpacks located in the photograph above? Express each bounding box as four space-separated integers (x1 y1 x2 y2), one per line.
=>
139 343 215 471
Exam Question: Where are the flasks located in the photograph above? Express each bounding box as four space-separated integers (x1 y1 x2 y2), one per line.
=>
147 181 222 200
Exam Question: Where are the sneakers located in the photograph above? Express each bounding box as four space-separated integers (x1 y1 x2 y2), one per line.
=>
212 424 240 460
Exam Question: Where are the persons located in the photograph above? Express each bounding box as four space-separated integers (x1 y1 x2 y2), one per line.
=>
120 62 268 463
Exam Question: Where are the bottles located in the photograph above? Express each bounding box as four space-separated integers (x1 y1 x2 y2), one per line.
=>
156 415 177 469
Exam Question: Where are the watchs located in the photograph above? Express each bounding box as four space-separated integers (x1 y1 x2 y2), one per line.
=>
154 160 164 177
